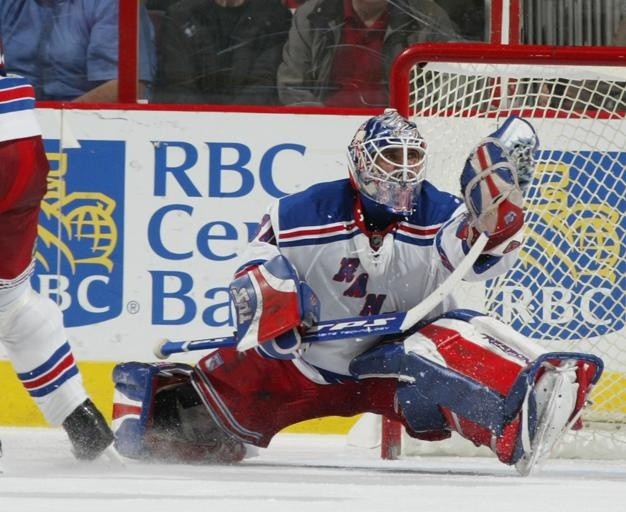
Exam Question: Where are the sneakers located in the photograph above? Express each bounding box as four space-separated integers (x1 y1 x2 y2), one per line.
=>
62 399 117 462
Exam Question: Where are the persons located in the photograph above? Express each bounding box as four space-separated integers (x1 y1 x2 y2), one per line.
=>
277 1 461 108
0 0 157 107
152 0 296 105
0 47 117 461
139 108 605 475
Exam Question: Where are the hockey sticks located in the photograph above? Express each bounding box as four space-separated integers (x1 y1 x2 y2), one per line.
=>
153 231 490 360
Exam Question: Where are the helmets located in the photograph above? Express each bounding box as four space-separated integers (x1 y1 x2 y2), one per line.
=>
346 108 428 218
